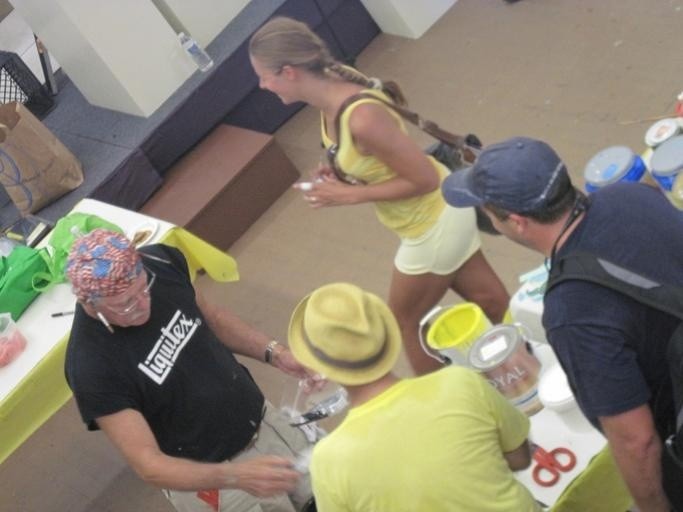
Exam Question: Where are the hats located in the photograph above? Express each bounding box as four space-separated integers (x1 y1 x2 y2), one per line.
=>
287 282 402 387
62 227 144 298
441 137 570 214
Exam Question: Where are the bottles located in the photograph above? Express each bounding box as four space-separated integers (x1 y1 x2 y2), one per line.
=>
175 31 214 73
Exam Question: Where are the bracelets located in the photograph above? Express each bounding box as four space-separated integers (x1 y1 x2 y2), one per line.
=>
263 340 278 364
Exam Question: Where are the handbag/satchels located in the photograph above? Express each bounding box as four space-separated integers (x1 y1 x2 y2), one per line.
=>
0 102 83 218
422 133 502 236
0 243 52 322
661 321 682 510
30 212 123 293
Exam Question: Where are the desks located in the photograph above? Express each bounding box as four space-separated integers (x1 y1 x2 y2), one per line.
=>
501 147 683 512
0 198 240 464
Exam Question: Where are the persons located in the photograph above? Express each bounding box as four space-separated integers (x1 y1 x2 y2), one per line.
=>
441 137 682 512
62 228 329 512
287 282 544 512
249 16 512 380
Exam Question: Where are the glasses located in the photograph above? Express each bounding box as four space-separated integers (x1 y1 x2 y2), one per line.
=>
94 263 156 317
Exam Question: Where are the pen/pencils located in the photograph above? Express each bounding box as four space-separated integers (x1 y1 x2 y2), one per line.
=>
51 311 76 317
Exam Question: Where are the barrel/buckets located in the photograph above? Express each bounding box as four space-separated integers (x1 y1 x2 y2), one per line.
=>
538 361 593 436
418 302 493 364
585 147 655 198
650 135 683 213
287 378 349 444
467 322 545 416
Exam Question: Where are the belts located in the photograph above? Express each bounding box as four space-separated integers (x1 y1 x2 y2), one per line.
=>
227 406 266 461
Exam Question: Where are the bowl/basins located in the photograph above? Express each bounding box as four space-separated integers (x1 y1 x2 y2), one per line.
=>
0 312 28 371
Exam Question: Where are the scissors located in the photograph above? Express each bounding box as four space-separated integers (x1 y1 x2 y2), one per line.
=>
521 440 578 485
